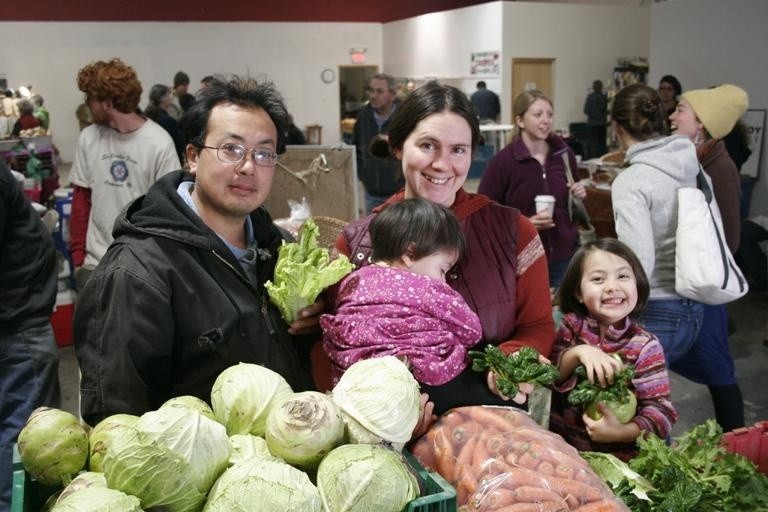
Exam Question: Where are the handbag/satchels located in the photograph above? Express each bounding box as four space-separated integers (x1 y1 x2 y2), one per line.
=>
562 151 598 251
674 171 750 306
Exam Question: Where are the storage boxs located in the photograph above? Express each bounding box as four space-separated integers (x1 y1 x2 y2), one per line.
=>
1 130 80 347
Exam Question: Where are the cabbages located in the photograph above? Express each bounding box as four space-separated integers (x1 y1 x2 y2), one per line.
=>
16 353 421 511
265 219 355 324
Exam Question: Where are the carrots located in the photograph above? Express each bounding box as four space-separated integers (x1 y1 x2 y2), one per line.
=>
412 405 630 512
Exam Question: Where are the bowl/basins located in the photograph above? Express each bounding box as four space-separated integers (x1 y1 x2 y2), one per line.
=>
0 140 20 153
20 136 52 150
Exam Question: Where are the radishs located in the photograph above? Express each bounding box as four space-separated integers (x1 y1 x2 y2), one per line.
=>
584 385 637 424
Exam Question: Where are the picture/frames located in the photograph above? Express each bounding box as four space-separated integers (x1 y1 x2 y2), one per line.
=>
735 107 767 179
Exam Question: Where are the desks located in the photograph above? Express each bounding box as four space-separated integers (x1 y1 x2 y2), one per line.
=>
471 124 518 151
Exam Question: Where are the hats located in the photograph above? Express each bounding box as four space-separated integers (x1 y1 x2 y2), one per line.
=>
661 75 681 94
680 84 750 140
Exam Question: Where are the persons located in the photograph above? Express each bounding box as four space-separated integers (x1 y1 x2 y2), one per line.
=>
0 59 323 512
314 58 751 459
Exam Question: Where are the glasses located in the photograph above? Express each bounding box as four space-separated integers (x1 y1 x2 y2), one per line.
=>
197 143 280 167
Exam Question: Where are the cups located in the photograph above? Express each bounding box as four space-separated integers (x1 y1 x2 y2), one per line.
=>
534 195 557 220
